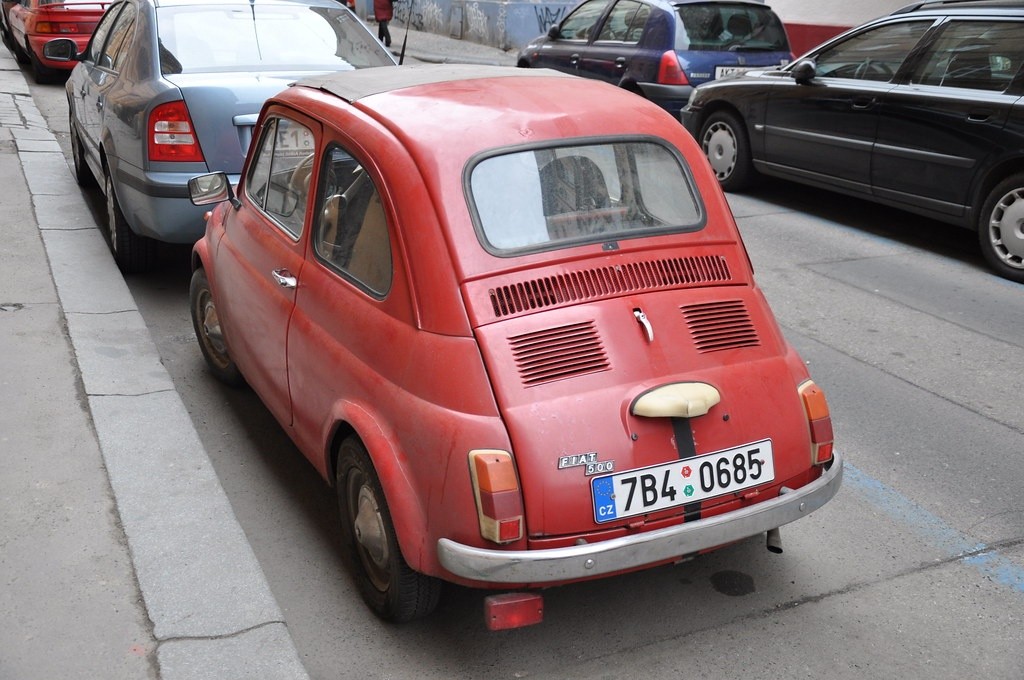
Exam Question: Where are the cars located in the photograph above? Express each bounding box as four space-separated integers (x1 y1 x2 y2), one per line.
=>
516 0 798 126
42 0 407 277
0 0 122 87
183 61 845 627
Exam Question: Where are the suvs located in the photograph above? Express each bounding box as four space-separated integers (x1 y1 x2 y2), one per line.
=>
678 0 1024 285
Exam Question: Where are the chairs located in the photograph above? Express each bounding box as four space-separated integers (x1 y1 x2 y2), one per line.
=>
722 13 752 51
620 9 649 42
939 38 993 90
520 154 612 244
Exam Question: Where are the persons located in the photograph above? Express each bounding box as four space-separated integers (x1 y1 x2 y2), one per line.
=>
374 0 393 47
336 0 356 13
